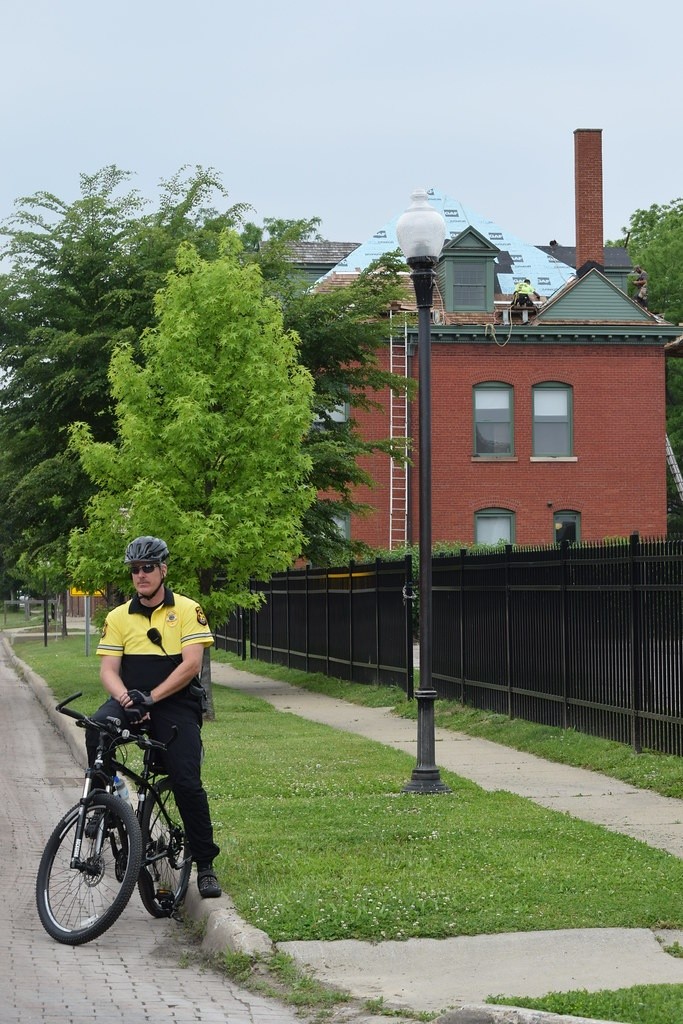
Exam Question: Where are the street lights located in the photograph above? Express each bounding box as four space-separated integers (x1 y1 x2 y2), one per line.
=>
393 184 454 794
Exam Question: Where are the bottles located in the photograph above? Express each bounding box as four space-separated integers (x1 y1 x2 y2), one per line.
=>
113 776 130 805
638 285 646 299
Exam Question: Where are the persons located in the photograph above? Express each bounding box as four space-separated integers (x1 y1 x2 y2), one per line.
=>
510 279 540 307
631 264 648 311
550 240 562 246
85 536 222 898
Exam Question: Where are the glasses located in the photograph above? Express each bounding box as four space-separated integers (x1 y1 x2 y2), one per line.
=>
130 564 158 574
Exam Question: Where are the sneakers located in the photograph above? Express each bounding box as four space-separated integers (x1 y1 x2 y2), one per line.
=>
86 809 102 836
197 869 221 895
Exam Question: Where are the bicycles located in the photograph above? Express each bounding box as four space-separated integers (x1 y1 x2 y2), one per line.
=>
34 693 195 947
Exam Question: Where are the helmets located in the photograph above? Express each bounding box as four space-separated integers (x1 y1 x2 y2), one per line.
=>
125 536 169 562
